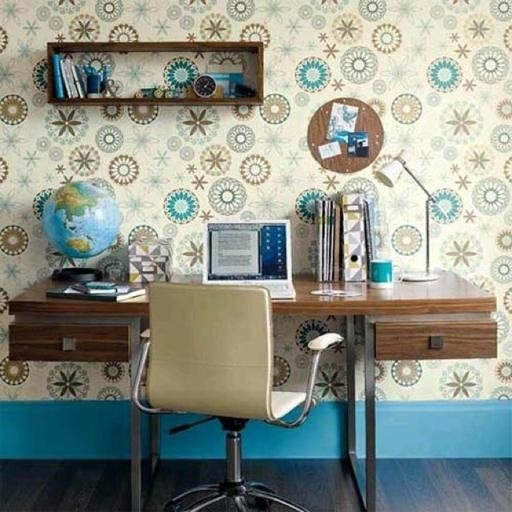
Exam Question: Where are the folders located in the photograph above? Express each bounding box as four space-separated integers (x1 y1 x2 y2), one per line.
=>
341 192 365 282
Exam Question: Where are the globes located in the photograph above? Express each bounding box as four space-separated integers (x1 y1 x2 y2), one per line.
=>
43 181 120 283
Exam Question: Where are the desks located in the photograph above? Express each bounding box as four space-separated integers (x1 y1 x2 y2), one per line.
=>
5 266 498 512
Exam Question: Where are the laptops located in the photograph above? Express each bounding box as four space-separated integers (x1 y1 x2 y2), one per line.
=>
202 219 296 301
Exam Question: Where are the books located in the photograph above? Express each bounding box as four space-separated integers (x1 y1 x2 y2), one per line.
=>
47 286 146 301
52 54 87 99
83 280 117 289
313 191 376 282
73 285 130 295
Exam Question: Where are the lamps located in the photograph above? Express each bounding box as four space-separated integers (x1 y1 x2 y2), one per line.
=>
375 149 452 283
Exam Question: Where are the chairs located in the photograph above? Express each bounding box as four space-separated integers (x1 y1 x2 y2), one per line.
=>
127 282 347 510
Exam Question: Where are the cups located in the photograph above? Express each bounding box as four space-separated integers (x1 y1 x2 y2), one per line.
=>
369 260 404 289
87 75 101 98
